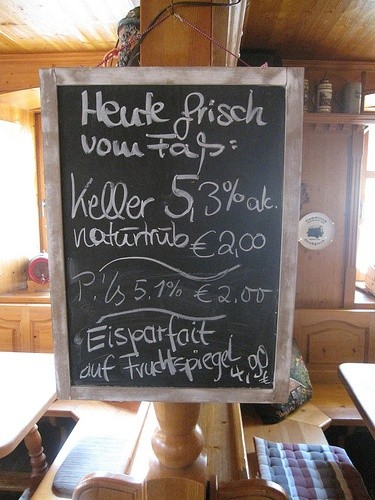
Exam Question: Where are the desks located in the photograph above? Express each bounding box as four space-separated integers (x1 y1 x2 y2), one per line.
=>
1 352 60 493
339 363 375 436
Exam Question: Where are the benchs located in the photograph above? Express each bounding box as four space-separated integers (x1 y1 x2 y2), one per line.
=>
1 303 206 499
206 308 375 500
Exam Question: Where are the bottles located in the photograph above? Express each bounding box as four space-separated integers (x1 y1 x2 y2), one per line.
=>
303 80 309 113
315 74 333 113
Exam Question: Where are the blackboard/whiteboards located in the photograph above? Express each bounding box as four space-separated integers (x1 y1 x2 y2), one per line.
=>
38 66 306 403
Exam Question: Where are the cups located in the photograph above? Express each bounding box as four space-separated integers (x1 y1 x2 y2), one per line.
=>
343 81 362 114
